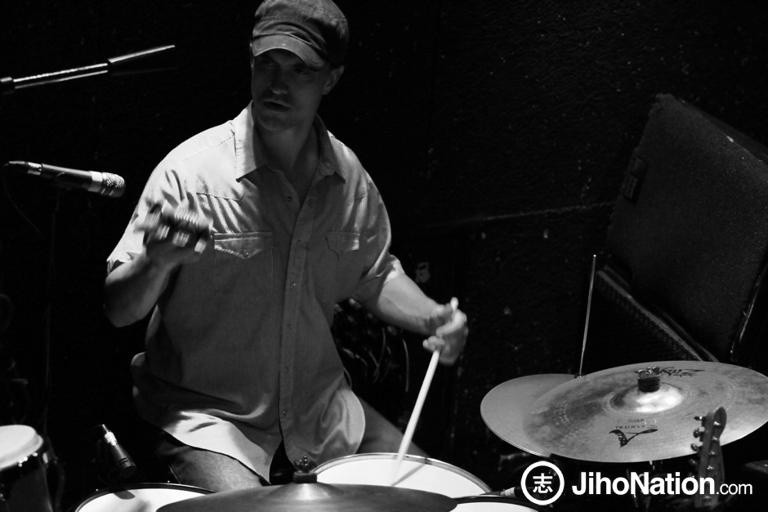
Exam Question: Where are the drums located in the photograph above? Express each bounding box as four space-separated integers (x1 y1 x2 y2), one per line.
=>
1 425 54 512
307 452 493 499
71 481 216 512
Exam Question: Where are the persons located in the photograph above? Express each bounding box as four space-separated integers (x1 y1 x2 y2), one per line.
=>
102 1 471 496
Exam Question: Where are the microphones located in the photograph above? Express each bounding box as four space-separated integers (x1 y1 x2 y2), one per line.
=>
7 159 127 201
101 423 138 475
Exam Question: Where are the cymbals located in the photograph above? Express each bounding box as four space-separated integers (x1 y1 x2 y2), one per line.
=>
156 482 457 510
479 359 768 463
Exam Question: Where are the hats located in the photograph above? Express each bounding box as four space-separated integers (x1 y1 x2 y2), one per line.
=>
250 0 349 75
